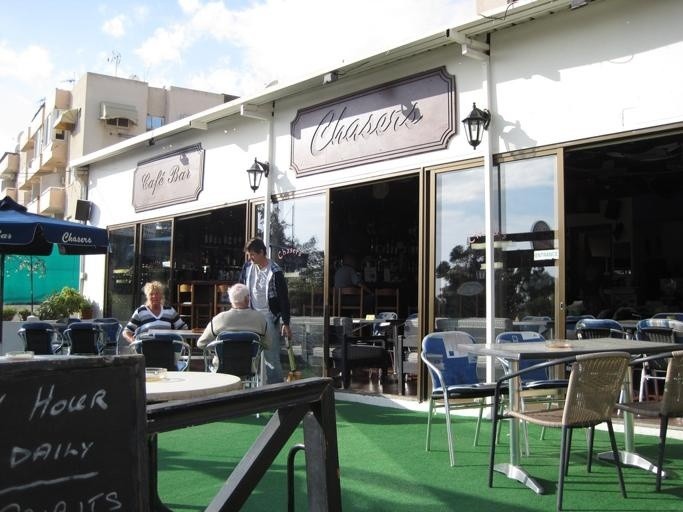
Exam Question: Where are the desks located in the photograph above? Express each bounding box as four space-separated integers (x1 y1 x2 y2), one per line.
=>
145 369 239 512
616 318 637 402
460 336 682 495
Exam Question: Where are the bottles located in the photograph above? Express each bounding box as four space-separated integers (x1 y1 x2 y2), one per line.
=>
329 239 418 273
113 263 166 288
202 230 245 282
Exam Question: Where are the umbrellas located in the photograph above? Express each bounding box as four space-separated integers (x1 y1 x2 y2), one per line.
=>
0 195 110 343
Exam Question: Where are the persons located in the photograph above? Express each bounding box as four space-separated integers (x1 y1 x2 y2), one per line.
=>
194 283 268 374
234 237 292 385
332 250 374 316
121 280 189 358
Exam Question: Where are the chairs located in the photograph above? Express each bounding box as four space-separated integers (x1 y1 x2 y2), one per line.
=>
634 328 682 418
495 330 568 456
586 350 682 492
636 317 682 331
575 328 630 341
488 351 630 511
613 306 640 320
420 330 530 466
574 318 621 330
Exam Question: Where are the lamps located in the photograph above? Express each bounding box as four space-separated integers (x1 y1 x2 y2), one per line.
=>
246 156 270 194
462 102 491 153
